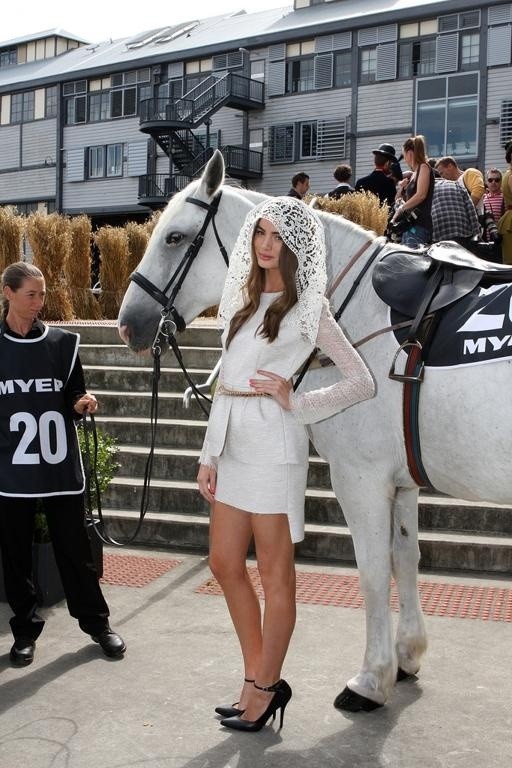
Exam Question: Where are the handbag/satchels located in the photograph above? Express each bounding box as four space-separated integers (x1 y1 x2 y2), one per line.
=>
389 199 420 232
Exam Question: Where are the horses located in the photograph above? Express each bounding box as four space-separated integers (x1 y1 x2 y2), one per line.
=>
118 148 512 712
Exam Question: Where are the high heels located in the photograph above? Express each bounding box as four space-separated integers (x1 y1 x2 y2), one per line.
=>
215 679 292 731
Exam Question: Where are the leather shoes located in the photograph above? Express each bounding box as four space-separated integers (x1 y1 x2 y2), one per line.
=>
92 628 125 657
10 642 35 665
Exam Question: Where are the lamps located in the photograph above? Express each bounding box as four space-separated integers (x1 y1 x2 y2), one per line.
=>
239 47 259 56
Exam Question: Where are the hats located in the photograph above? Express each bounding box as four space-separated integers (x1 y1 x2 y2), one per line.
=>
373 144 399 162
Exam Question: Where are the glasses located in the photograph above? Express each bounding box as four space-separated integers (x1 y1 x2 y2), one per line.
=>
488 178 500 183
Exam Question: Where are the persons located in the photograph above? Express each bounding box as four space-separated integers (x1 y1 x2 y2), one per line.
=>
287 136 512 264
196 197 377 732
0 262 127 666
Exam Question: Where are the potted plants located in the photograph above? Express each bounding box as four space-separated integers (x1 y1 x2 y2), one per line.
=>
29 422 121 610
